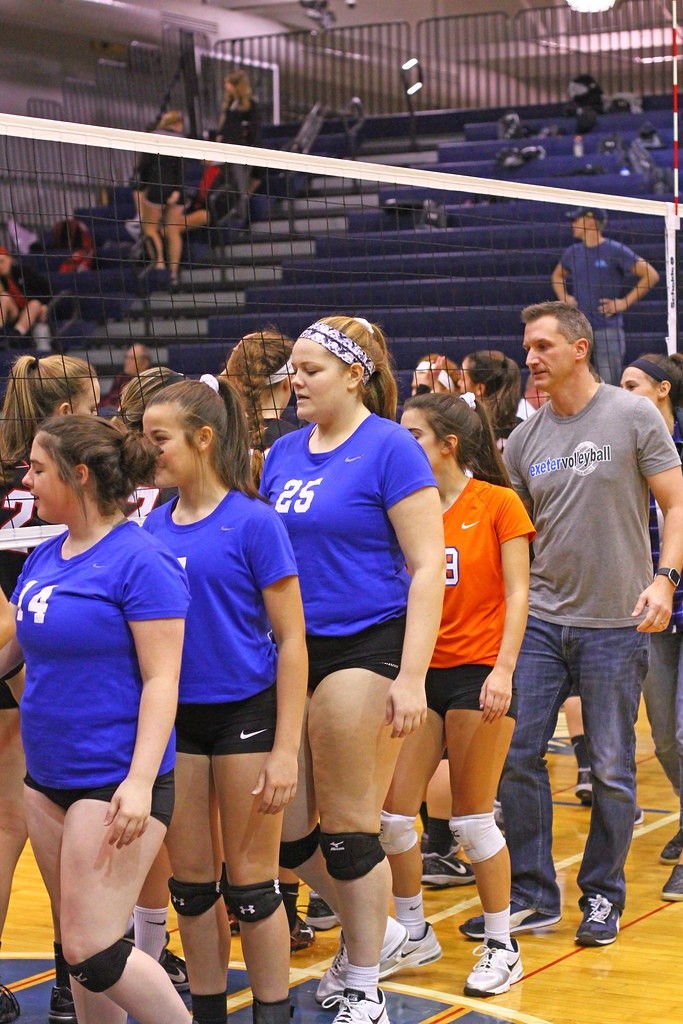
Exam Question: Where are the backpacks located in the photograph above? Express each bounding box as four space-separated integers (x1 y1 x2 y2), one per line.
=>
499 108 524 140
569 74 603 116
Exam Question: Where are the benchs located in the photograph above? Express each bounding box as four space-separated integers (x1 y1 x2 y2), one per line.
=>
0 92 683 423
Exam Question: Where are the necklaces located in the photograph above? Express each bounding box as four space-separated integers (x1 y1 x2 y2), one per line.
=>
310 424 319 439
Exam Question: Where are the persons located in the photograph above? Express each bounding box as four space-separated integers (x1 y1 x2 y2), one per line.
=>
0 69 270 356
549 207 660 386
1 298 683 1024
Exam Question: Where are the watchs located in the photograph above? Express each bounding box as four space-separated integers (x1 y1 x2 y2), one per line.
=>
653 567 681 586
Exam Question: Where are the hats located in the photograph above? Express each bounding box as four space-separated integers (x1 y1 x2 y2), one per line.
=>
565 206 607 220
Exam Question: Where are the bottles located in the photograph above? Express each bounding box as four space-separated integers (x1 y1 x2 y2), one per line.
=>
573 136 584 157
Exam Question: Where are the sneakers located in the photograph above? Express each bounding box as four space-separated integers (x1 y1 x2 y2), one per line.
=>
464 937 524 997
574 894 620 946
315 915 410 1006
459 900 562 939
421 832 477 887
377 922 443 981
322 987 391 1024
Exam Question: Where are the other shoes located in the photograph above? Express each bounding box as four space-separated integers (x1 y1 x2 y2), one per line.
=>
659 829 683 902
152 268 181 293
223 898 241 935
122 924 190 991
130 240 150 265
60 250 101 273
0 986 78 1024
634 805 644 824
493 799 506 836
305 891 341 930
0 332 23 351
290 905 315 953
575 766 593 804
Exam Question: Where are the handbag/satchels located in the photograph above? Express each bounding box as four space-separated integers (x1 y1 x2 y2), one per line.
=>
575 93 676 195
51 218 93 249
492 145 546 177
418 199 448 229
